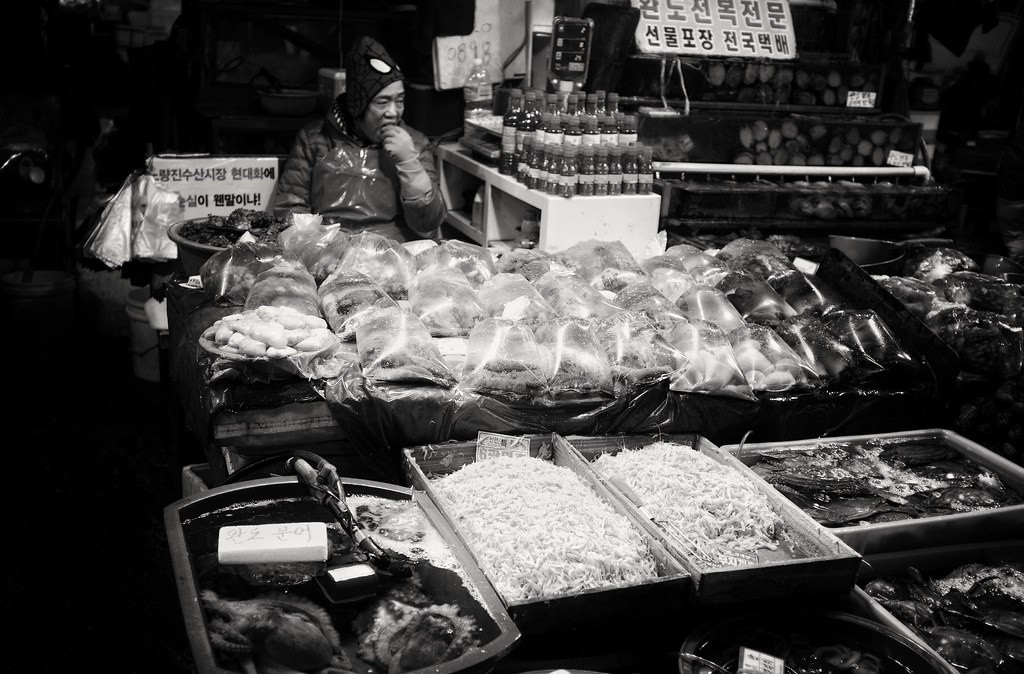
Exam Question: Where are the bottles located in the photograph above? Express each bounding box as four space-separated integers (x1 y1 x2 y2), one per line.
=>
463 57 493 138
500 87 654 198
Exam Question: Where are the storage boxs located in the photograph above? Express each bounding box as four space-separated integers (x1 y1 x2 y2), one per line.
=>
158 234 1024 674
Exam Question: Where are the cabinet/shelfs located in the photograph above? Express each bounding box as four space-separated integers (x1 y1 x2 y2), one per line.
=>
616 49 949 242
430 144 663 253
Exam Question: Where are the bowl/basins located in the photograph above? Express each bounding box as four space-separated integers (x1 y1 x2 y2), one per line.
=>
678 610 954 673
168 217 230 276
831 237 907 278
982 253 1021 278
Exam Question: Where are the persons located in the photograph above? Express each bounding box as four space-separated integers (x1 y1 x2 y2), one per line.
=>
273 35 447 245
